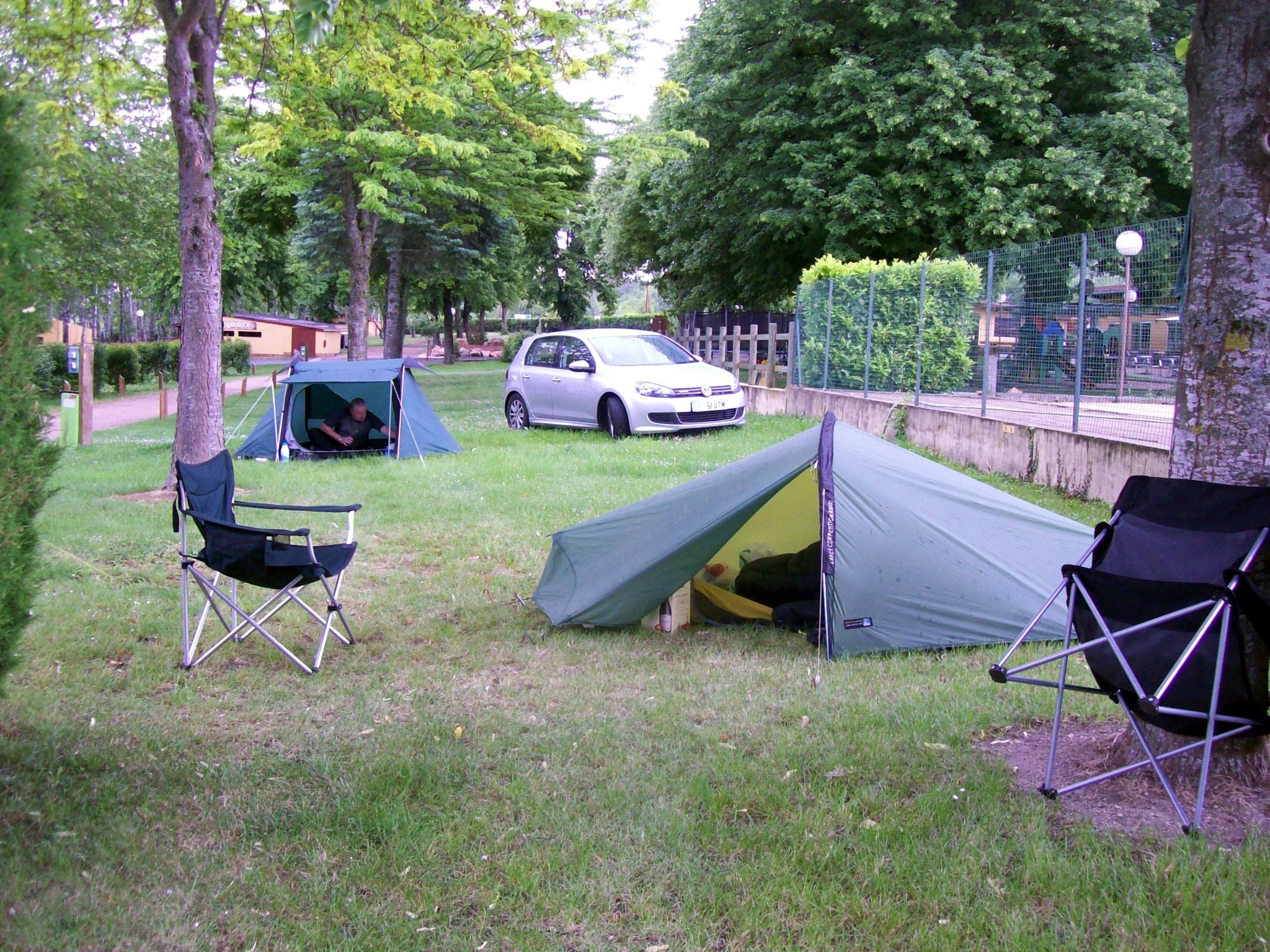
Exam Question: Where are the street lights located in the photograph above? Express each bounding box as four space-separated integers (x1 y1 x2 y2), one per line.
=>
643 272 653 313
1115 230 1143 402
1123 289 1137 352
136 309 145 342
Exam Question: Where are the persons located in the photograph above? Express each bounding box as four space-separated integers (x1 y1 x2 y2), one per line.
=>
308 398 398 451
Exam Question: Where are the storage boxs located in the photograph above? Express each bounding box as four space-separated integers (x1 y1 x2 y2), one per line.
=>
640 579 691 634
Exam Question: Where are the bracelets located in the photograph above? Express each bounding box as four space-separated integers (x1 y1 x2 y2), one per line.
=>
339 438 341 441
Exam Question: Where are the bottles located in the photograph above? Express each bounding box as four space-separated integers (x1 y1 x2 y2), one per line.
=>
280 440 290 462
659 598 672 633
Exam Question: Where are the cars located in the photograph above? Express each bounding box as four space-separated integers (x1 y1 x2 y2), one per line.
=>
504 328 745 441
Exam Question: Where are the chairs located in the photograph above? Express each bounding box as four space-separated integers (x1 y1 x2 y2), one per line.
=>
988 475 1270 840
172 449 362 676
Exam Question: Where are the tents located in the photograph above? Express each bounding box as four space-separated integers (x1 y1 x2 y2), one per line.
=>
532 411 1094 660
225 355 463 469
